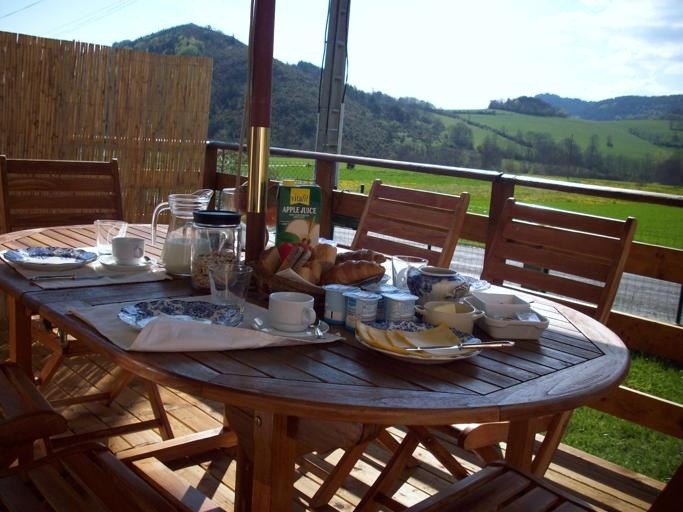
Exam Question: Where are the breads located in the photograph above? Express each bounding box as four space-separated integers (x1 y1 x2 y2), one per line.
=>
260 243 386 285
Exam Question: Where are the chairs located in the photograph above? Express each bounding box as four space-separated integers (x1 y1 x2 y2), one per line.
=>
1 154 682 512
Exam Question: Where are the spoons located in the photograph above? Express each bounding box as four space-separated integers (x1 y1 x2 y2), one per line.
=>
308 318 324 340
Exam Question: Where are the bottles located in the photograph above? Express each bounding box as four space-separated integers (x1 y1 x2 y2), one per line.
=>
214 186 246 261
407 265 472 303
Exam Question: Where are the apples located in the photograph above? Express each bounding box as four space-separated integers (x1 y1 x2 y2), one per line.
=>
285 215 320 246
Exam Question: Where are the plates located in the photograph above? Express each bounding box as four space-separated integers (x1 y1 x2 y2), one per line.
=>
356 318 485 365
3 246 95 270
250 312 329 341
459 273 492 294
120 298 243 334
98 254 153 273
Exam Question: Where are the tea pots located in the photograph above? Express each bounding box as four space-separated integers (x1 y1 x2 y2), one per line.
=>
150 187 214 278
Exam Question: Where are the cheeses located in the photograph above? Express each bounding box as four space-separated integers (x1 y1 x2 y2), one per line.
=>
355 319 462 357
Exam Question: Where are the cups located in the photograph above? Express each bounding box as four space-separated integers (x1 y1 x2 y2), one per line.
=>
111 237 146 266
413 298 485 335
203 264 253 314
92 219 131 256
267 290 316 333
322 252 430 334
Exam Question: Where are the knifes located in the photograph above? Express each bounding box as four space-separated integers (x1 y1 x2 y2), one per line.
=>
399 340 517 352
31 274 125 282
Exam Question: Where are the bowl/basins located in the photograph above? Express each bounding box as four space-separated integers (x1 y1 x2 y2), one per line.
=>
255 245 392 295
